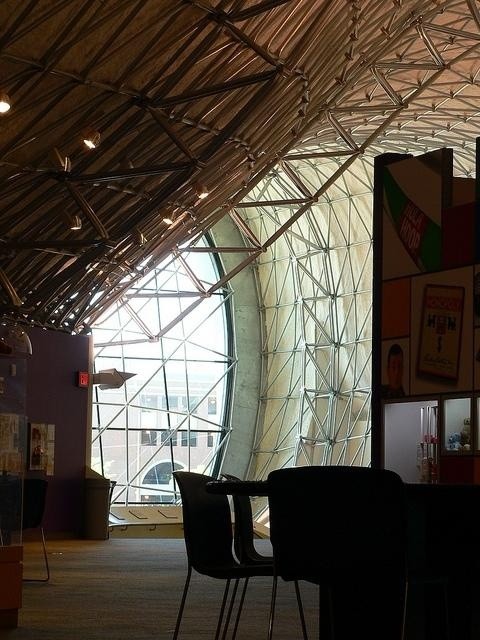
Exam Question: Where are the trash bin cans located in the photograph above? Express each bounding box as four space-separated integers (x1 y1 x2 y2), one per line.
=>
86 465 110 540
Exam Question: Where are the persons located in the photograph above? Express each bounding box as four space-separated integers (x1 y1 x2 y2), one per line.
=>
382 344 406 399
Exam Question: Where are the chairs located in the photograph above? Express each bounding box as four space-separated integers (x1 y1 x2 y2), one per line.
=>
0 478 49 583
172 471 309 640
218 474 307 640
316 466 451 639
268 467 320 639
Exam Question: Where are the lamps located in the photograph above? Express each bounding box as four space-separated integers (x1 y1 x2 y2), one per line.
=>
189 173 209 200
68 208 84 231
82 126 101 150
0 84 11 114
157 205 175 225
129 226 147 248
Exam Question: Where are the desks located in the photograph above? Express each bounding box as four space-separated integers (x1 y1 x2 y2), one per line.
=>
204 480 480 640
0 545 23 631
